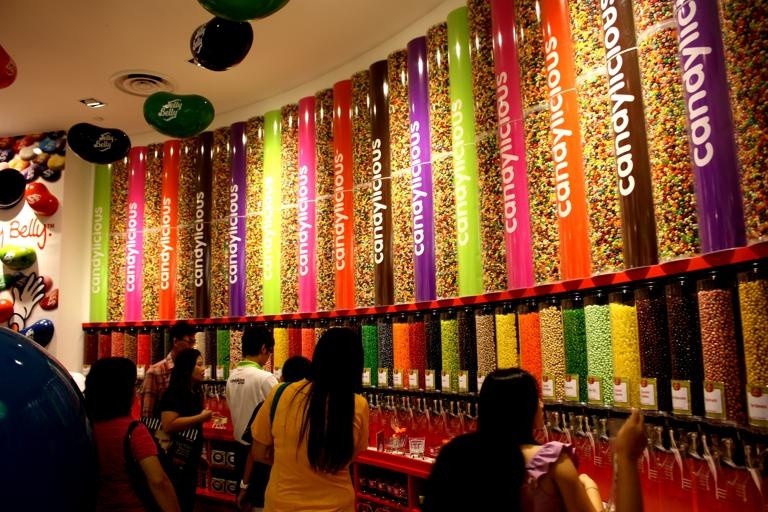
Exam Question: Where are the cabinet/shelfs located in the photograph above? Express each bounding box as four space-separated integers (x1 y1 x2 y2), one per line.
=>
194 426 433 512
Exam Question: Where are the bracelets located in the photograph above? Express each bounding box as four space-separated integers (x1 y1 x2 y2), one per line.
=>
238 479 250 490
582 485 601 493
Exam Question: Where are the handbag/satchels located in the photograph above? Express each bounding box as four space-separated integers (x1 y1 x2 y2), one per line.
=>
124 416 203 512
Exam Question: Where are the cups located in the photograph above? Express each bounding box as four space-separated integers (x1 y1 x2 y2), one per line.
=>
212 416 228 430
385 436 425 462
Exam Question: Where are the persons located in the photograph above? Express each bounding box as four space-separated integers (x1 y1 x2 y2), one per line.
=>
139 320 196 420
473 365 605 512
218 325 279 511
150 347 214 511
606 406 649 512
77 353 181 512
231 353 313 511
247 325 371 511
414 430 523 512
152 428 173 456
